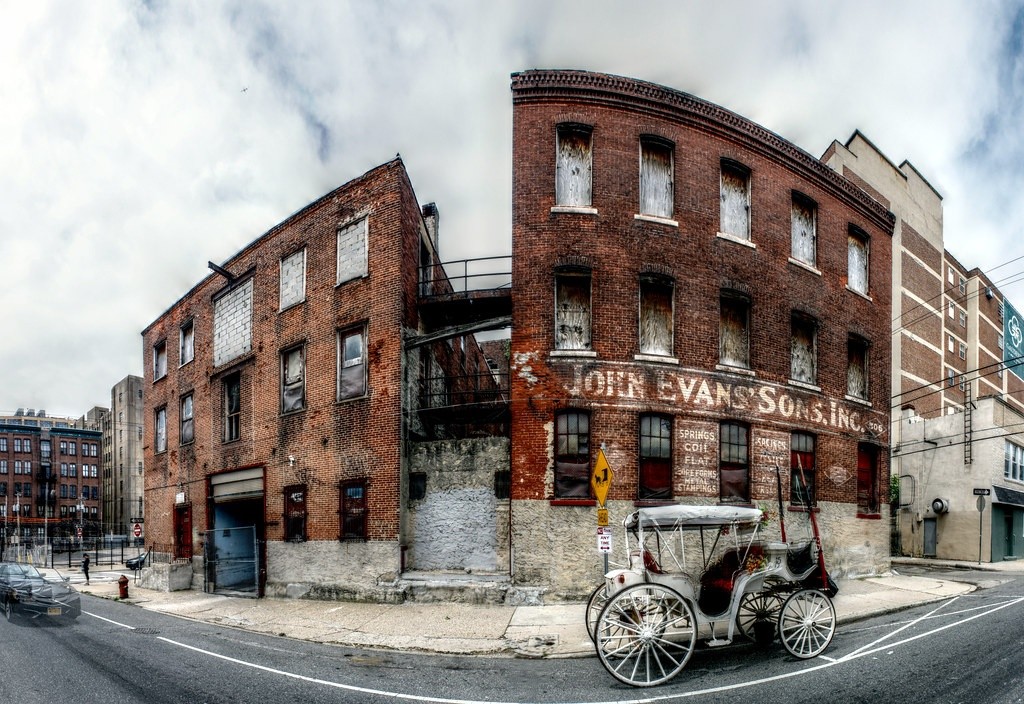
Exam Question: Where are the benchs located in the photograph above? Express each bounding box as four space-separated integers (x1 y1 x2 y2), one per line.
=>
642 549 664 574
700 546 765 592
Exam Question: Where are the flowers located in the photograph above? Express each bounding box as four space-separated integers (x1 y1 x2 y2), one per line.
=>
718 523 730 535
757 506 768 531
746 553 769 576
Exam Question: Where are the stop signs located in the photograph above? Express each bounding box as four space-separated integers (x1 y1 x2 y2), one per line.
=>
77 528 82 537
133 524 142 538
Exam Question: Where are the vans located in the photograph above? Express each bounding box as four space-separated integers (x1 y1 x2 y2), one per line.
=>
126 552 147 571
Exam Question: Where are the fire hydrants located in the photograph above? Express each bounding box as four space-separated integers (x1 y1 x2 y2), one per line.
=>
117 574 130 599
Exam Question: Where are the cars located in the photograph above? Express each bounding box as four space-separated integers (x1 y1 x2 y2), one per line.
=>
0 560 82 627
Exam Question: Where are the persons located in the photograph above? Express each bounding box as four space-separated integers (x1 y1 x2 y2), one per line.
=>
80 553 91 585
